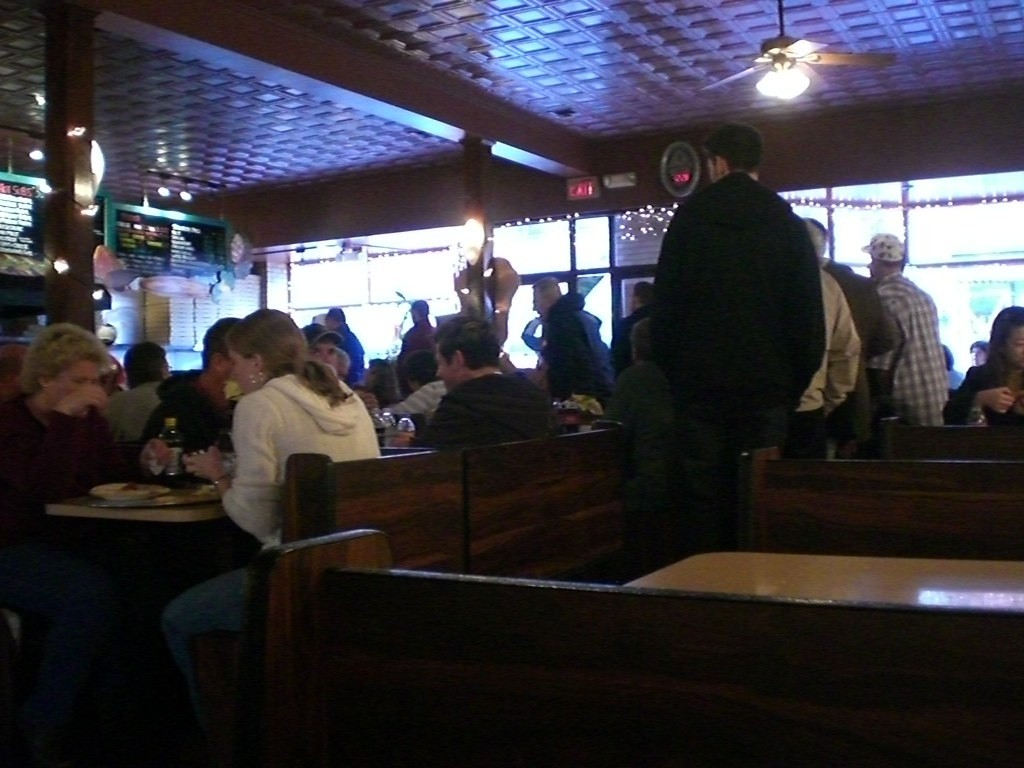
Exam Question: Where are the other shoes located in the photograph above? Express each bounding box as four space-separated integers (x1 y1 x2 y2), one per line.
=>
17 707 71 767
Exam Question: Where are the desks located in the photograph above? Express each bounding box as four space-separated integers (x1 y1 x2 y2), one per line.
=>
620 551 1024 611
43 497 227 522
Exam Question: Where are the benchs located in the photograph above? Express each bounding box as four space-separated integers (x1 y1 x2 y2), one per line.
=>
189 412 1023 768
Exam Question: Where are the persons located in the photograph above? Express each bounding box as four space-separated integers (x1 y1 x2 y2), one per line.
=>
161 310 382 768
601 219 1024 584
99 275 656 486
0 324 171 768
651 121 827 561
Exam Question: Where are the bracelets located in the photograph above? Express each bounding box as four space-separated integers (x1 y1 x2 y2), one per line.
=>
210 473 234 484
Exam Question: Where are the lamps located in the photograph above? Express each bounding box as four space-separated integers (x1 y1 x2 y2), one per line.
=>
756 55 810 98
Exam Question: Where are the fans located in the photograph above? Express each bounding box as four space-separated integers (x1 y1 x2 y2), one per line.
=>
700 0 897 91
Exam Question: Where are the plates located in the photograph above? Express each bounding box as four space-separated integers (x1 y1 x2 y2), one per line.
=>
90 483 171 500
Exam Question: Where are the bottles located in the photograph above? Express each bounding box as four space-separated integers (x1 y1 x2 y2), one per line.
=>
397 413 416 431
380 407 396 428
552 398 562 408
966 406 987 425
157 418 187 488
370 408 386 447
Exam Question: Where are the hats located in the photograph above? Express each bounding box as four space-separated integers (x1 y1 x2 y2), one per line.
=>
861 233 905 261
302 323 345 345
